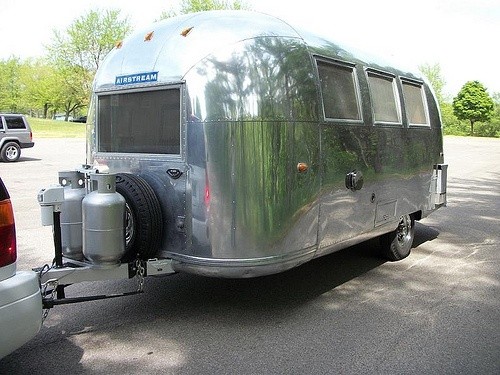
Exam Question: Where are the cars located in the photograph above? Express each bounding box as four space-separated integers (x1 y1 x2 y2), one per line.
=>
52 114 73 121
69 115 87 124
0 178 45 366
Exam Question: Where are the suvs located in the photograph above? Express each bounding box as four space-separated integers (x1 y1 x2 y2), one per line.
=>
0 112 36 164
